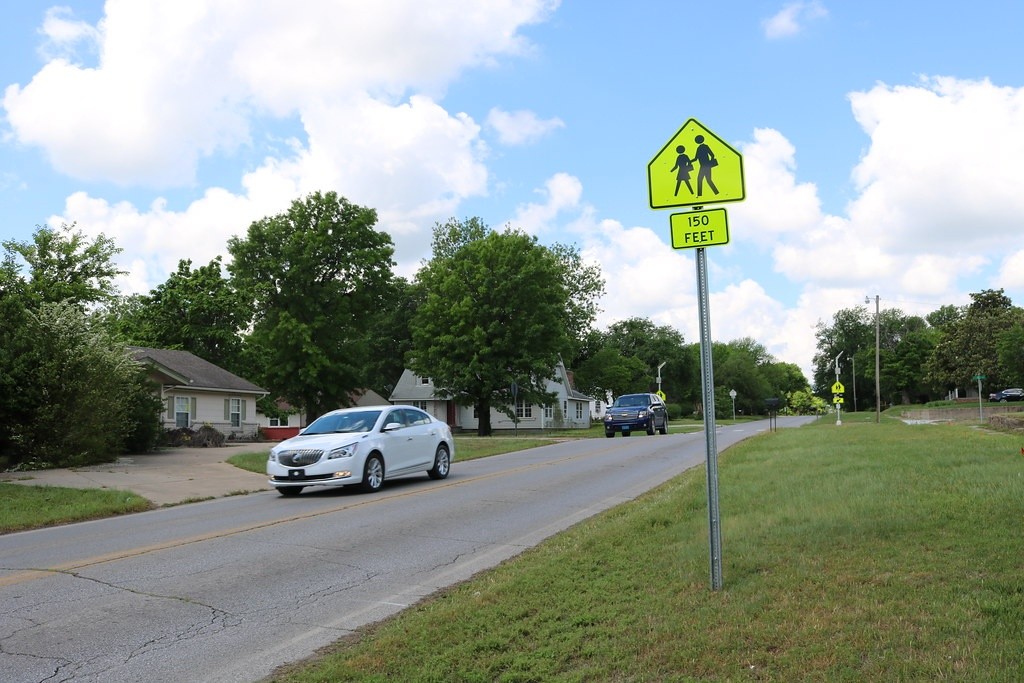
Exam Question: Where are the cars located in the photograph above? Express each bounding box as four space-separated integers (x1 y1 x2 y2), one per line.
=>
988 388 1024 403
265 403 456 495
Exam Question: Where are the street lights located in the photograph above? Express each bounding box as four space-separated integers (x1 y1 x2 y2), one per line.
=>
865 295 880 423
846 357 857 412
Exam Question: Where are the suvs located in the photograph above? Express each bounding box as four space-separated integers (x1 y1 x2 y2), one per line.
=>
602 392 669 435
